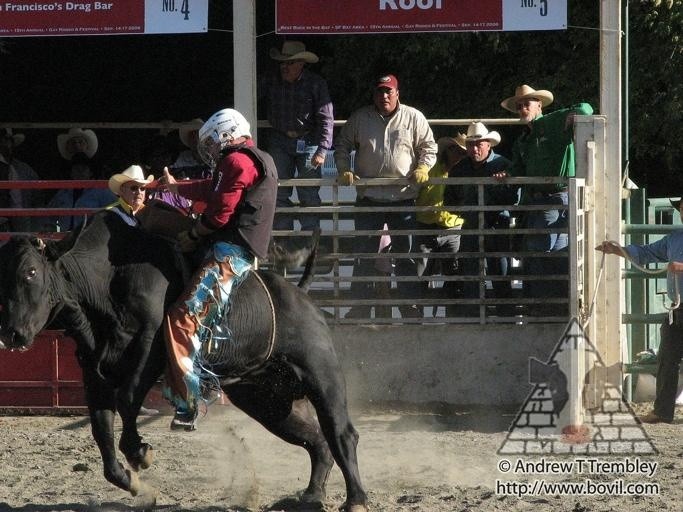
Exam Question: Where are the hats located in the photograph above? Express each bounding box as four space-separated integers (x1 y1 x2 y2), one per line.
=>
669 193 683 210
437 134 468 158
270 40 319 64
179 118 205 148
369 74 399 94
458 121 501 149
0 128 26 148
109 165 155 195
57 127 99 160
500 85 554 113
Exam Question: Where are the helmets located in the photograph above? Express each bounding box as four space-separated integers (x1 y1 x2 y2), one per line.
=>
197 108 251 168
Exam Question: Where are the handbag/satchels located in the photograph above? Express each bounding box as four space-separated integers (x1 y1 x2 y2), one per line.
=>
135 198 196 244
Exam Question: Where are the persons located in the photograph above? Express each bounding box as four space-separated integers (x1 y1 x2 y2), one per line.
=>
442 120 518 326
333 70 439 325
409 127 467 290
42 152 117 259
257 39 334 256
156 102 278 431
489 82 595 317
0 123 45 248
594 196 683 424
167 116 205 169
57 127 122 177
100 161 156 220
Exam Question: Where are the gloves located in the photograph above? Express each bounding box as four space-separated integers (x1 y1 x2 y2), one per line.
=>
337 168 360 187
409 164 430 184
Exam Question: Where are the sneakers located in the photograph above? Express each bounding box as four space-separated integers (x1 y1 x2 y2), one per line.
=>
498 305 517 323
641 409 672 424
401 305 424 324
169 408 198 431
345 306 371 324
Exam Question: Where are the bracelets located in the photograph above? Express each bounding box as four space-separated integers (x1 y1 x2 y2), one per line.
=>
185 228 202 244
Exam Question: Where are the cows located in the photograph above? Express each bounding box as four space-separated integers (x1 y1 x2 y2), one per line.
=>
0 200 367 512
